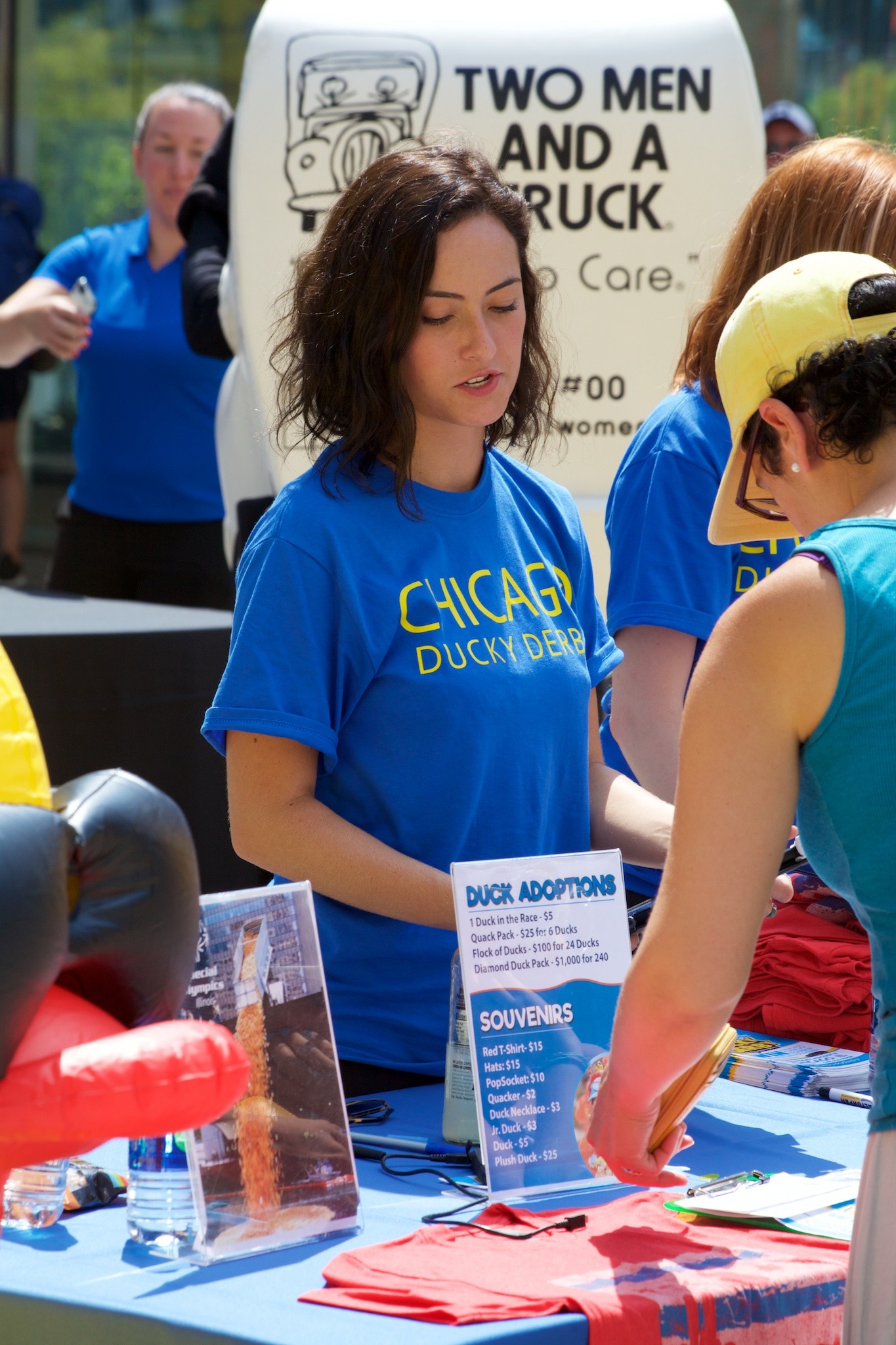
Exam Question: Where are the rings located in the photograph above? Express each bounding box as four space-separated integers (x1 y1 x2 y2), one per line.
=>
622 1166 644 1181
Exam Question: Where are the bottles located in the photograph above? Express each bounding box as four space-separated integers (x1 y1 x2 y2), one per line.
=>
0 1156 69 1231
127 1136 198 1251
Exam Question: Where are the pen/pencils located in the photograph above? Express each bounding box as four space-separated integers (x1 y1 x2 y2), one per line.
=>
351 1131 466 1155
353 1144 386 1160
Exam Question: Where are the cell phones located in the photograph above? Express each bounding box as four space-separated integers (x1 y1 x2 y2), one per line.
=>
68 277 94 316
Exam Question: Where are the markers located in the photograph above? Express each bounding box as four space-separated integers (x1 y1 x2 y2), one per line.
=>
818 1086 875 1109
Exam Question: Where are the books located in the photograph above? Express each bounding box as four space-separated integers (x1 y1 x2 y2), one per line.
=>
716 1010 871 1101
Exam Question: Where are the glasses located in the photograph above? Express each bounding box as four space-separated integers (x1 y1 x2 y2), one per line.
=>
736 402 810 522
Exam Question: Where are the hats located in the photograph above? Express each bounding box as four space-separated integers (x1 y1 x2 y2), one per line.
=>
761 99 819 139
707 251 895 546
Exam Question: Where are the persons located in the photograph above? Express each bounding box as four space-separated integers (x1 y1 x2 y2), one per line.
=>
762 99 817 191
597 131 895 921
0 81 236 616
585 251 895 1345
200 143 800 1101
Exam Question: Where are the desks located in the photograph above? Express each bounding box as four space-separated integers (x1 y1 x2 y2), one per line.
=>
0 1078 872 1345
0 585 268 896
